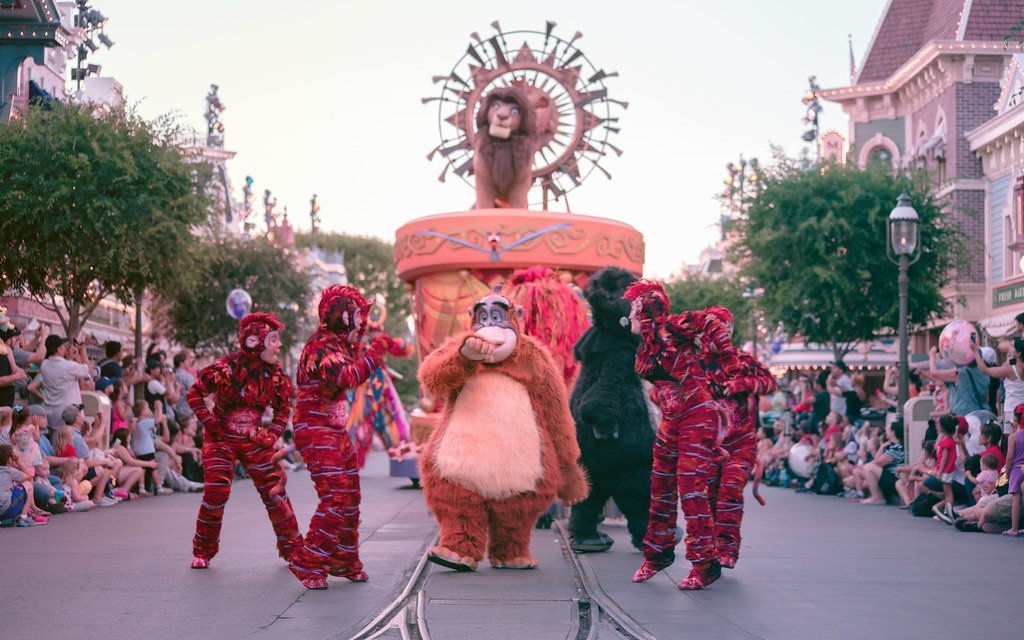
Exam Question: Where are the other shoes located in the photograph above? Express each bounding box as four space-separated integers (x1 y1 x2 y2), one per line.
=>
47 504 65 513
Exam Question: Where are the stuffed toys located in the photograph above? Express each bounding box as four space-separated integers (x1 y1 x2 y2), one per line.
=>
417 294 590 574
567 264 683 590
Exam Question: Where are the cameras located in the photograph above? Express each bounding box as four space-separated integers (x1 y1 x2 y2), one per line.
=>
96 413 101 418
935 348 940 352
72 338 78 347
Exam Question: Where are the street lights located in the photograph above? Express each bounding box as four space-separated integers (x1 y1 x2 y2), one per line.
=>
741 276 759 359
887 189 922 424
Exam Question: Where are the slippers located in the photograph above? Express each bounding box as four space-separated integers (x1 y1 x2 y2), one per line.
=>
1002 530 1019 537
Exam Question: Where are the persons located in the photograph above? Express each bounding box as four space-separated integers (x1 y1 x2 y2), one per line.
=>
621 279 778 592
0 305 307 526
748 312 1024 537
185 283 394 589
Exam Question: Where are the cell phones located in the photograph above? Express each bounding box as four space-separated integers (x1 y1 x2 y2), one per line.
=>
956 424 959 438
971 332 976 344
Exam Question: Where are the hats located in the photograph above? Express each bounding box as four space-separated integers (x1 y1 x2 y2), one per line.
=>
28 405 52 416
62 403 85 425
45 335 71 355
95 376 117 390
0 326 21 342
829 360 846 373
147 359 165 368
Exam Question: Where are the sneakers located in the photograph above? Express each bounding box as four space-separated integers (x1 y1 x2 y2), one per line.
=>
71 500 94 512
139 491 153 497
346 570 369 582
91 496 122 507
677 561 722 589
17 509 52 527
301 577 329 589
837 488 887 505
156 488 174 495
191 556 210 568
632 552 675 582
953 518 1001 534
795 482 812 493
191 483 204 492
717 555 737 569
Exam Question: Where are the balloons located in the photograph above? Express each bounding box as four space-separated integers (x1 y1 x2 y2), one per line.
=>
225 289 253 318
